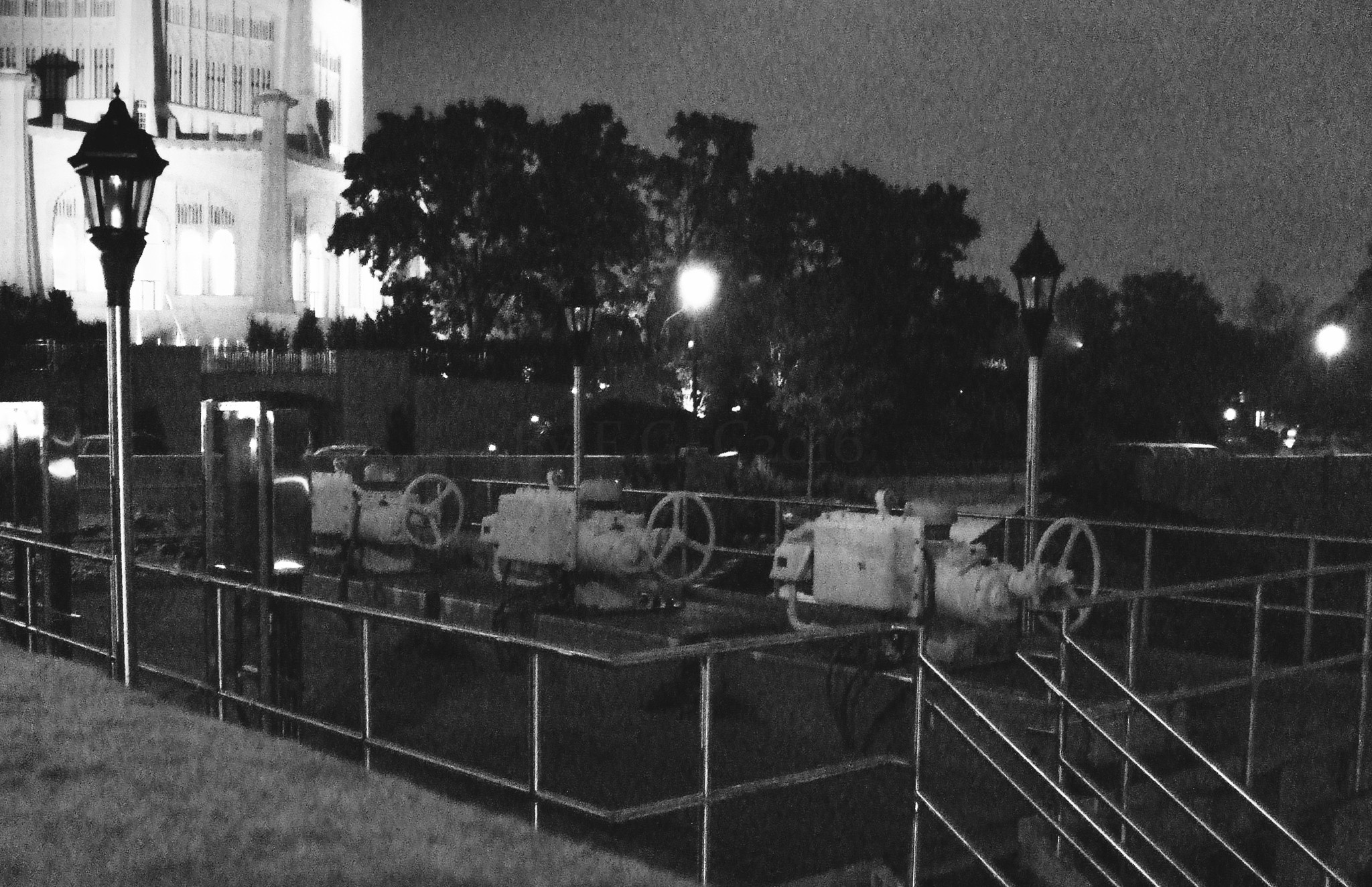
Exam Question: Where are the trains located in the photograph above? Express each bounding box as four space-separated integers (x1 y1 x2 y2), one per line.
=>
476 472 716 611
303 442 464 580
768 489 1102 679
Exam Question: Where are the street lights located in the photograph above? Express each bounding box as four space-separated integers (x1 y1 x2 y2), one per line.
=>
561 272 596 490
1009 216 1067 635
678 266 717 416
67 83 172 694
